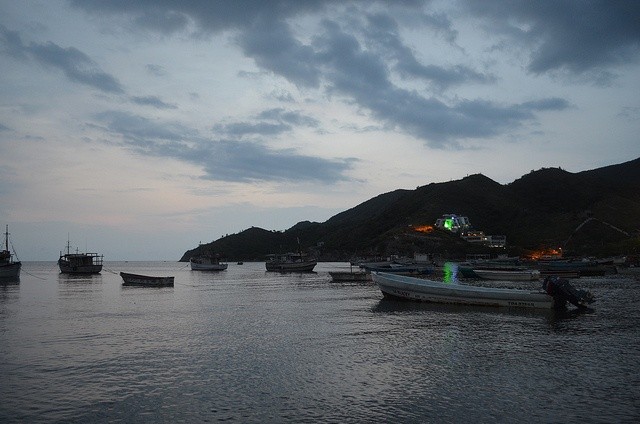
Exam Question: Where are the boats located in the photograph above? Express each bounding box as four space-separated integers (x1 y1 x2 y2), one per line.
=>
473 270 540 281
328 270 433 281
266 252 316 272
120 272 174 287
190 250 227 271
371 271 595 309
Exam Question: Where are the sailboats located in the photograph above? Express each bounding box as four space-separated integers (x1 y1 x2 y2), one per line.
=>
0 225 22 282
57 234 104 273
